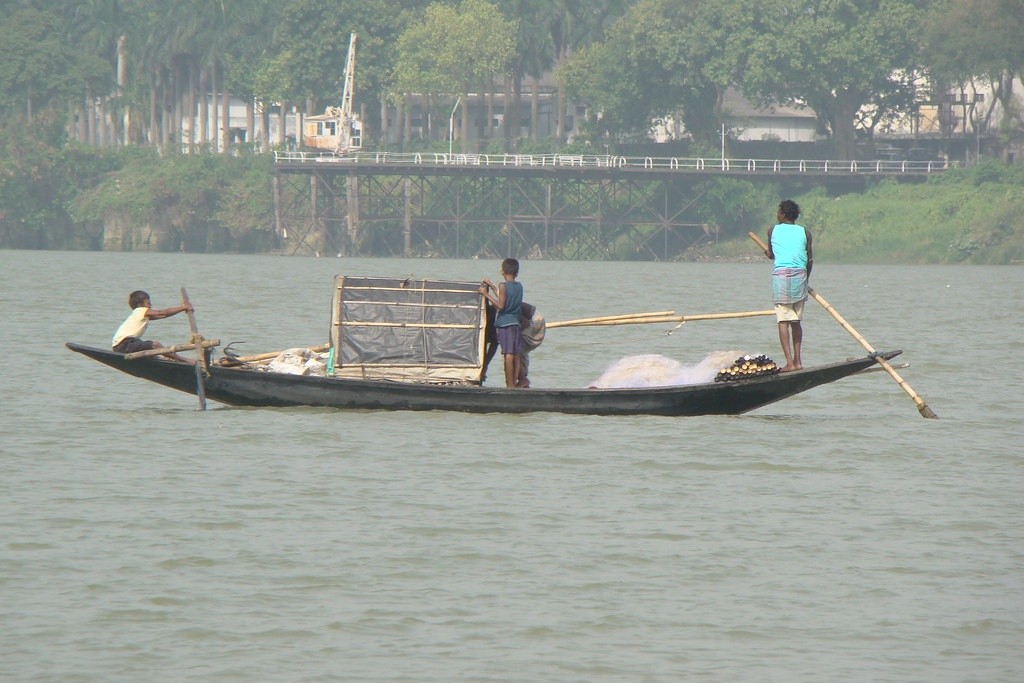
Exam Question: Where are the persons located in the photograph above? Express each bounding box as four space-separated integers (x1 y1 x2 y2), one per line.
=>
112 291 195 362
765 200 813 372
478 258 546 388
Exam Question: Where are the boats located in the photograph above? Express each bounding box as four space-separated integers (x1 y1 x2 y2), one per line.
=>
63 277 906 415
